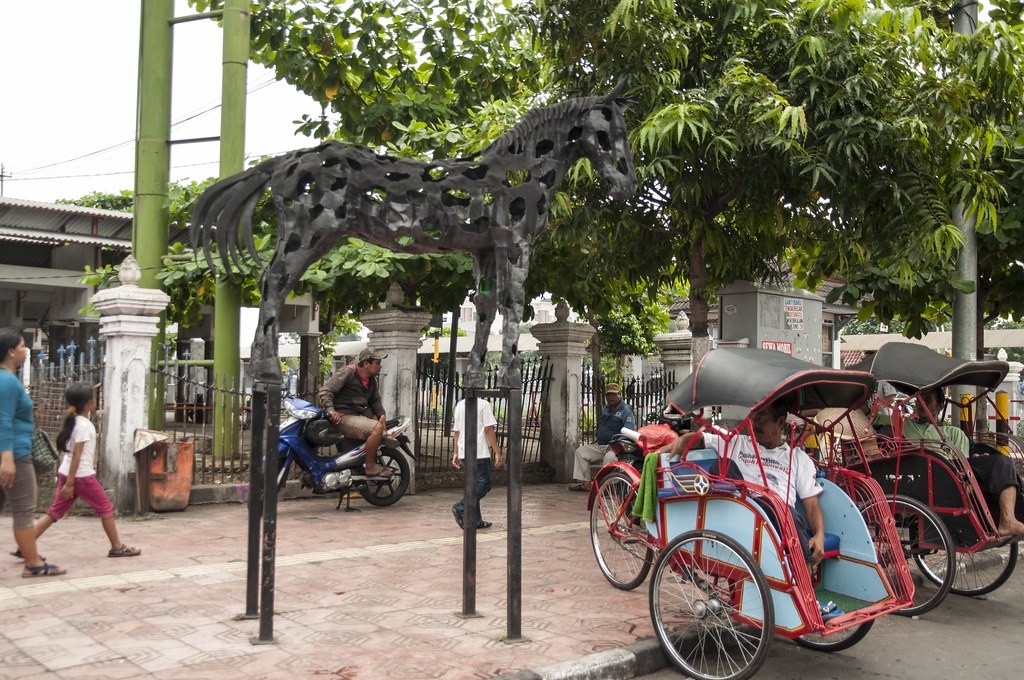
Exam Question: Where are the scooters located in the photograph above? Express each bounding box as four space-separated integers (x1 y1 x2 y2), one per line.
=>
246 384 421 511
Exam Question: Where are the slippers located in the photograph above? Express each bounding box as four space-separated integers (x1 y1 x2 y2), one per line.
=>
10 548 46 560
818 600 845 620
108 544 141 556
366 466 395 477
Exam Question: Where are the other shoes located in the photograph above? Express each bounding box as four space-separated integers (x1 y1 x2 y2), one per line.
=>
603 491 619 498
568 480 592 491
452 507 464 531
478 520 492 528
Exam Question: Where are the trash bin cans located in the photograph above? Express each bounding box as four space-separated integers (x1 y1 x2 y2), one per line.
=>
147 441 196 512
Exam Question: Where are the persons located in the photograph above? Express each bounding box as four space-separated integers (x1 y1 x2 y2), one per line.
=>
9 381 142 561
317 348 396 478
568 382 636 492
0 327 68 578
451 398 504 530
858 384 1024 536
653 401 845 623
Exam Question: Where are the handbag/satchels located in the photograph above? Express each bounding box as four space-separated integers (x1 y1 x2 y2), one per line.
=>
30 412 58 475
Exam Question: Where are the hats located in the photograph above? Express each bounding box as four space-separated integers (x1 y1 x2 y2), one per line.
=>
358 347 388 360
604 382 620 395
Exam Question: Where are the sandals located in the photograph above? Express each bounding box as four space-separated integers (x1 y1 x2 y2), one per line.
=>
22 561 66 577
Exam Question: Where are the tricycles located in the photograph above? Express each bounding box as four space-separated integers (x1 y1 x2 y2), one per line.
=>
585 345 918 680
806 342 1023 618
